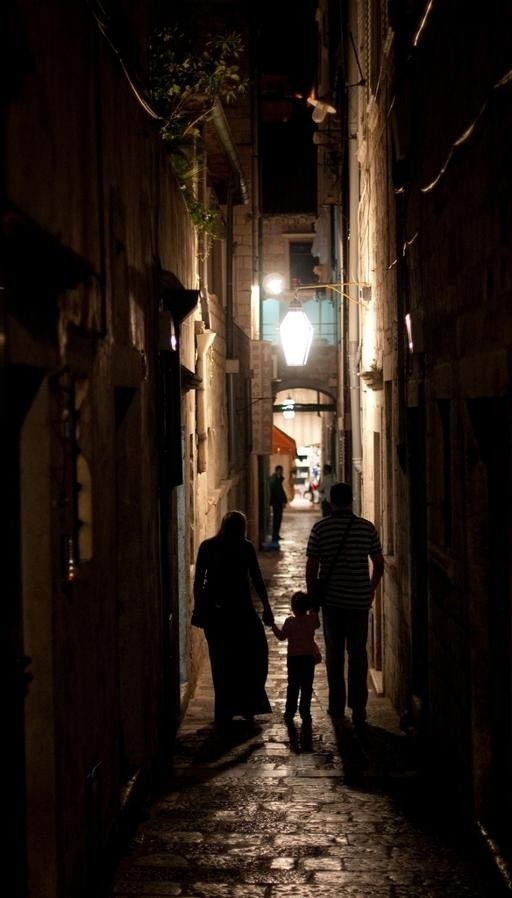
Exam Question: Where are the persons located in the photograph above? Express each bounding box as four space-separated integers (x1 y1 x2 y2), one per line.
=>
191 510 274 734
304 482 385 718
267 465 288 540
317 463 336 517
272 591 320 721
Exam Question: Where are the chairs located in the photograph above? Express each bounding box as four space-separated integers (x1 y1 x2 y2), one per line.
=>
327 707 345 720
352 707 367 725
282 711 312 722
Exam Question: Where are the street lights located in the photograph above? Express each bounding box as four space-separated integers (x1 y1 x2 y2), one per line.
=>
191 588 207 628
318 569 328 598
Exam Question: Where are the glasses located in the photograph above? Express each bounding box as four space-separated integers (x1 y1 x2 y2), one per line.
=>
279 282 369 367
308 77 366 124
